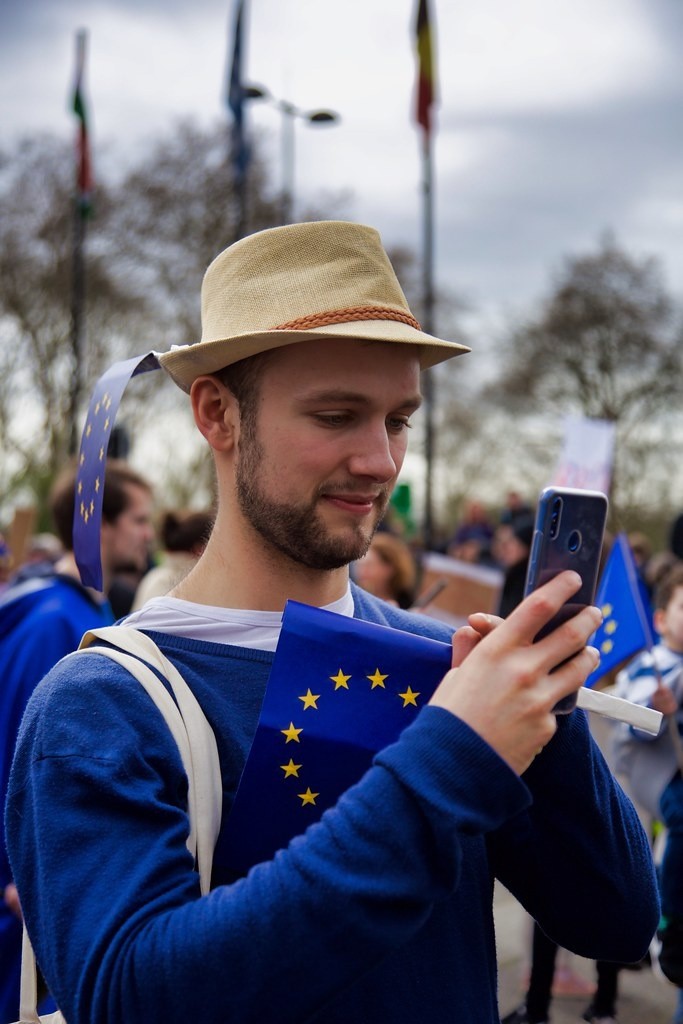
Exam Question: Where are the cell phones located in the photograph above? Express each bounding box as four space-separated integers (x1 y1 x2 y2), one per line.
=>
521 484 609 715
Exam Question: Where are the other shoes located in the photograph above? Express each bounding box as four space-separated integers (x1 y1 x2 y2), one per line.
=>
502 1007 551 1024
580 1003 619 1024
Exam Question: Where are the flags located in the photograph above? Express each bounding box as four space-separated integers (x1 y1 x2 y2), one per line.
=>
226 2 248 187
209 600 452 892
76 352 159 591
584 534 658 687
70 79 89 216
414 0 434 129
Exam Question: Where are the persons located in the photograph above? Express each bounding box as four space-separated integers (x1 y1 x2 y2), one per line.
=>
0 461 153 1024
129 490 683 1024
7 223 662 1024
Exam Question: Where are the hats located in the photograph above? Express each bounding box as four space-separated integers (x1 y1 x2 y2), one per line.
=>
72 220 473 593
